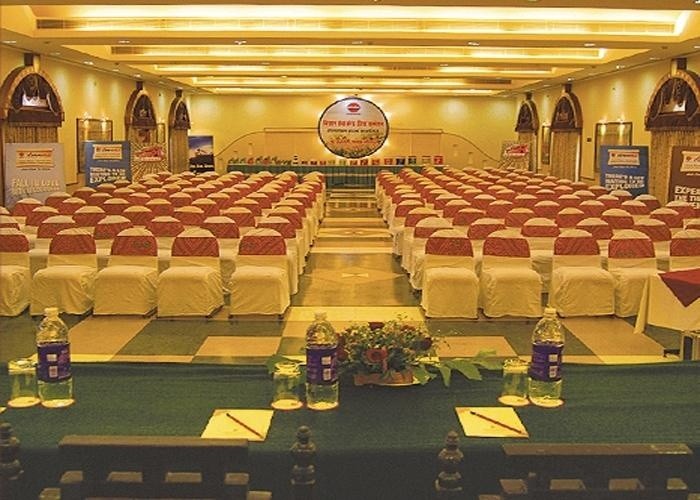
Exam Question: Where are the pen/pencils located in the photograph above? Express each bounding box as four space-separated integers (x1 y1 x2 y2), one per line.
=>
470 411 521 434
226 413 261 436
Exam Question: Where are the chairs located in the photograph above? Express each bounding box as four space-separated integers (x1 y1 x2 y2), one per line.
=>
375 166 700 321
2 171 326 321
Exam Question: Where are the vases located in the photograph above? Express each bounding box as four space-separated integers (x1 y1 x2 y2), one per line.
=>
366 365 417 388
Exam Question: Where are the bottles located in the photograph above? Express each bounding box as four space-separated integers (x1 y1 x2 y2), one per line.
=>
531 306 565 408
34 304 75 409
304 308 340 412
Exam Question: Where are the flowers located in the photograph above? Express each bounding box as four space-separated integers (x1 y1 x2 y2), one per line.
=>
333 313 502 386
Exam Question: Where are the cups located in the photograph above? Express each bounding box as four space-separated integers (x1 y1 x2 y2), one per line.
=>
6 357 41 409
497 358 531 407
271 361 303 410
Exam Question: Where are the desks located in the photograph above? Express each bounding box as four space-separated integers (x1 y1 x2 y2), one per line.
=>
226 163 450 186
0 358 700 500
